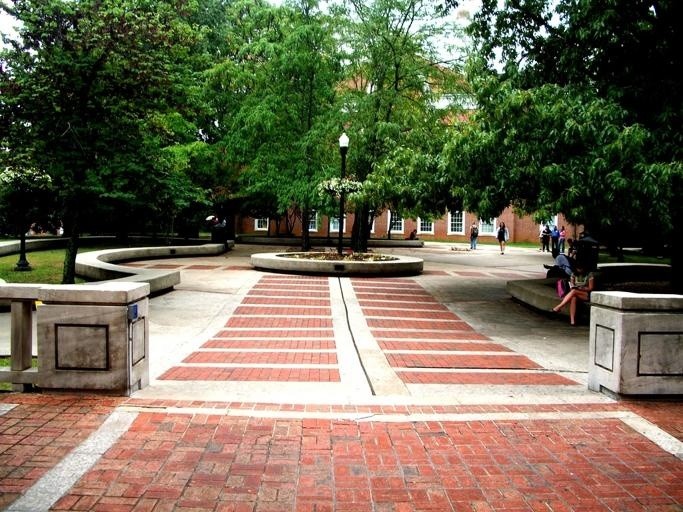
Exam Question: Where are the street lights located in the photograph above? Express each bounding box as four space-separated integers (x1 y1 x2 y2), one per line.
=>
338 133 350 256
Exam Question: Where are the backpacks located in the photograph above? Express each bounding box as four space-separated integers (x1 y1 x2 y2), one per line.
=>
560 254 577 272
471 226 478 238
557 279 570 298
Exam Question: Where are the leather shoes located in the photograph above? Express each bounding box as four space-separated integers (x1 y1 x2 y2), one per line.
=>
549 309 561 313
570 324 576 327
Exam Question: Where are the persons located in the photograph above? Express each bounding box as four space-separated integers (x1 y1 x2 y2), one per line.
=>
496 221 509 255
549 265 601 326
470 221 478 250
539 223 577 278
409 229 419 240
